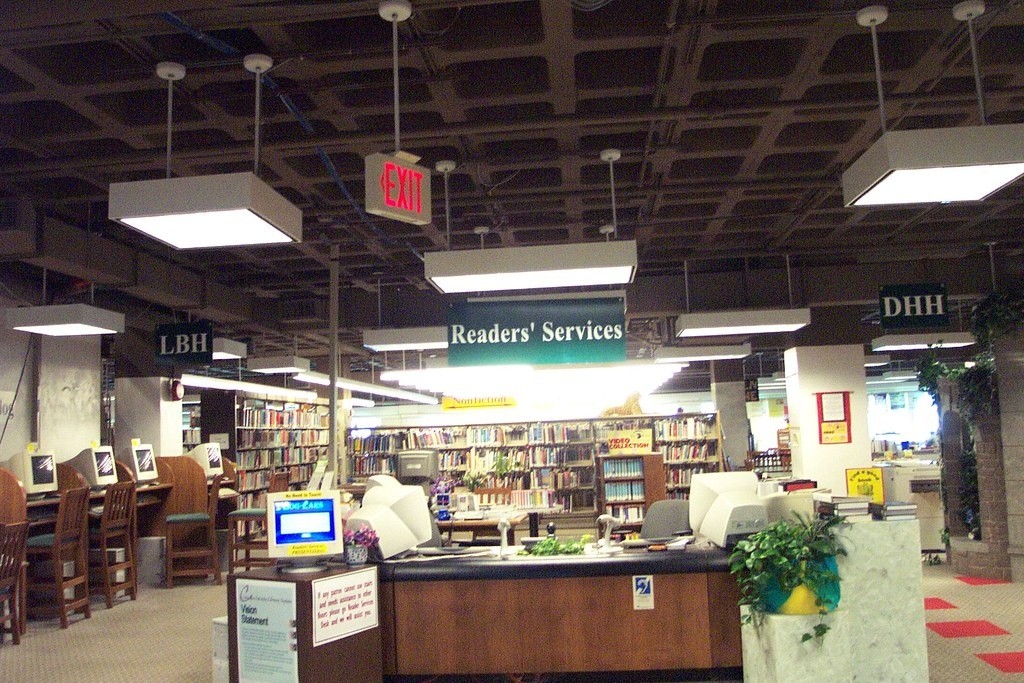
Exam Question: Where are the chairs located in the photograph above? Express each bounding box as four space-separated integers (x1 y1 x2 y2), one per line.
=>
0 517 31 646
640 499 690 539
164 473 225 589
227 470 291 575
89 480 137 609
472 484 512 541
26 485 91 629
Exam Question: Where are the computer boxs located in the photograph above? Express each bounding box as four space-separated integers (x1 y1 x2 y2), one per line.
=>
135 536 168 587
26 561 76 619
89 549 126 601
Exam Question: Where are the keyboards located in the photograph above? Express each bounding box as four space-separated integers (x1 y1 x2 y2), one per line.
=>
218 487 236 495
92 501 124 513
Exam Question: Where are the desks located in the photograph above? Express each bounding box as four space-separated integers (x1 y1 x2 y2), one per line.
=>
337 482 366 495
484 503 565 537
436 514 529 546
0 457 238 635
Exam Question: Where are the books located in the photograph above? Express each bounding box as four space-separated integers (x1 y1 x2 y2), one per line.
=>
752 447 792 473
182 429 200 453
237 399 331 536
872 438 897 455
759 477 917 525
344 415 735 543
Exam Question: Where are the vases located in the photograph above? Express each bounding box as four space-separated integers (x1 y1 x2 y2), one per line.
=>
435 493 449 507
437 510 451 521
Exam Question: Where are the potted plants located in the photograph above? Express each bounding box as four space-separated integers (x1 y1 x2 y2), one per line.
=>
727 507 858 646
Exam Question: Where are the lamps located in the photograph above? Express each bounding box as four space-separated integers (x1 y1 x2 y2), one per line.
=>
248 334 311 374
423 148 639 296
362 270 449 352
212 338 247 360
651 343 752 365
292 336 439 404
674 252 811 338
107 53 304 251
883 360 917 380
871 285 977 352
864 354 891 367
843 0 1024 209
755 351 786 390
5 265 126 338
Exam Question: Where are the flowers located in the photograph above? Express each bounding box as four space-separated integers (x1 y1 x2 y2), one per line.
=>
430 476 464 497
344 545 368 569
343 522 379 549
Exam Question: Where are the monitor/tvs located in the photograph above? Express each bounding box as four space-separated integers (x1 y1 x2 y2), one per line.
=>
0 451 58 501
64 445 118 495
347 485 433 560
689 471 770 550
182 442 223 485
115 444 158 489
398 451 439 498
267 490 344 574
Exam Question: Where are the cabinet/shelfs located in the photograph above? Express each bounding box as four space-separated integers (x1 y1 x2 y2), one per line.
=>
595 451 668 539
346 410 726 532
199 389 331 541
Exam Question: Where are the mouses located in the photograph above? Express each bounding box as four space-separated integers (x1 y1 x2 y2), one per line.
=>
222 476 229 481
150 481 160 486
